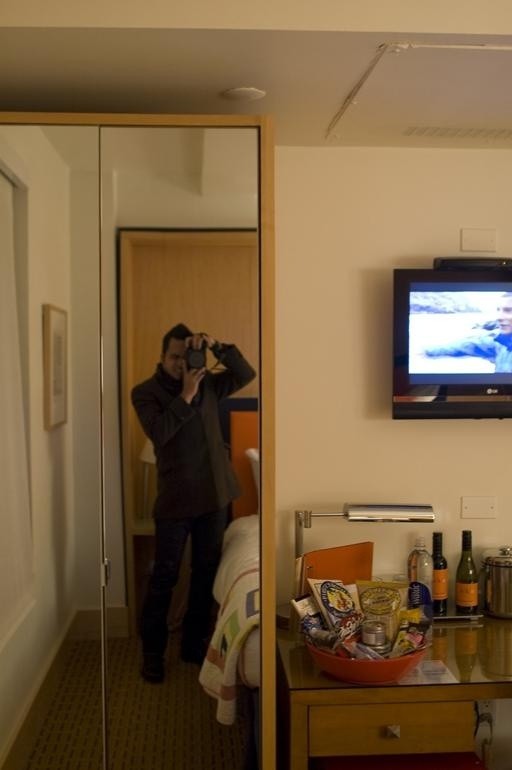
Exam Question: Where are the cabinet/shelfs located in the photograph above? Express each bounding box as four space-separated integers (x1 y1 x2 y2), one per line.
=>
277 596 512 770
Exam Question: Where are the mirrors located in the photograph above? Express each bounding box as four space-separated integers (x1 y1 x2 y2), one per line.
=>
101 122 260 769
3 123 102 769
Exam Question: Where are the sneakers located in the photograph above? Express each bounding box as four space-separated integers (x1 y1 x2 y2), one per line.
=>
142 655 163 682
181 643 207 663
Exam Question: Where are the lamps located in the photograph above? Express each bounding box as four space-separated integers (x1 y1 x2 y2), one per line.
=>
278 502 437 628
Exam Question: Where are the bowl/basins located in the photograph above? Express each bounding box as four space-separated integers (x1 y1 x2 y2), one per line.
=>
303 639 433 686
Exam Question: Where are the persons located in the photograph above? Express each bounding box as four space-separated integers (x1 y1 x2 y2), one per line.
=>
129 321 257 686
418 293 511 374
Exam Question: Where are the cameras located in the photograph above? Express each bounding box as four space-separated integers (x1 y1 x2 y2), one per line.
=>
186 340 206 368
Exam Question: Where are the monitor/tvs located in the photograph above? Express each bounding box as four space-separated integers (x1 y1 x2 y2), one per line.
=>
391 267 512 418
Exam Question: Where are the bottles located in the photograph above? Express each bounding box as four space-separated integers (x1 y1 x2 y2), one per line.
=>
405 526 483 616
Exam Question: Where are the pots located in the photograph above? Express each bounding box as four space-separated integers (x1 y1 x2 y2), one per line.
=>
480 544 512 619
